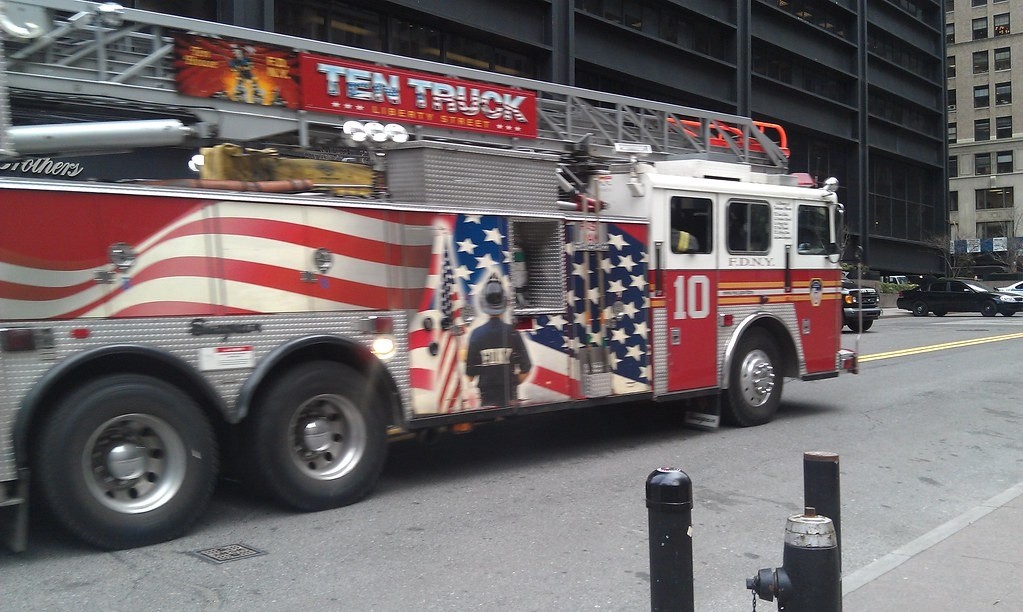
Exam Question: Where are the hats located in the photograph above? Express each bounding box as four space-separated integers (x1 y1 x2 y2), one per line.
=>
478 282 508 315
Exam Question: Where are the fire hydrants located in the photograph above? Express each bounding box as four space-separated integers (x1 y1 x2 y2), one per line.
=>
743 506 843 612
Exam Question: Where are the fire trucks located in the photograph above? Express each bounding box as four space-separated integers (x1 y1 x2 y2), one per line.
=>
0 2 864 553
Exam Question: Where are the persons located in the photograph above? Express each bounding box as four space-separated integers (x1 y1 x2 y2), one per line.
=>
672 196 700 252
464 278 531 408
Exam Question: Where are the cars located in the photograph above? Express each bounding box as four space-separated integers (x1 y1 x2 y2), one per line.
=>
840 271 882 332
994 280 1023 293
896 277 1023 318
